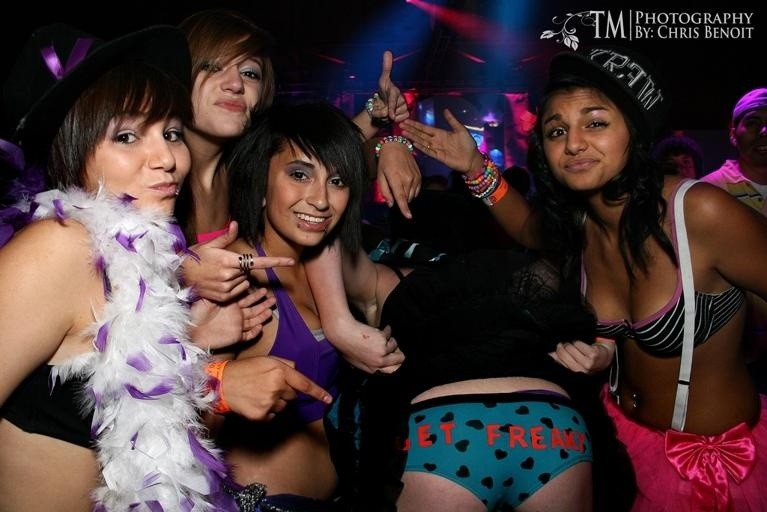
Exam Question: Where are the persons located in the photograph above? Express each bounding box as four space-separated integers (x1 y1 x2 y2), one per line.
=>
3 12 764 512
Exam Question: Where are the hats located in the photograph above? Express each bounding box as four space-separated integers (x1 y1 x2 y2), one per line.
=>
733 88 767 122
546 41 669 129
